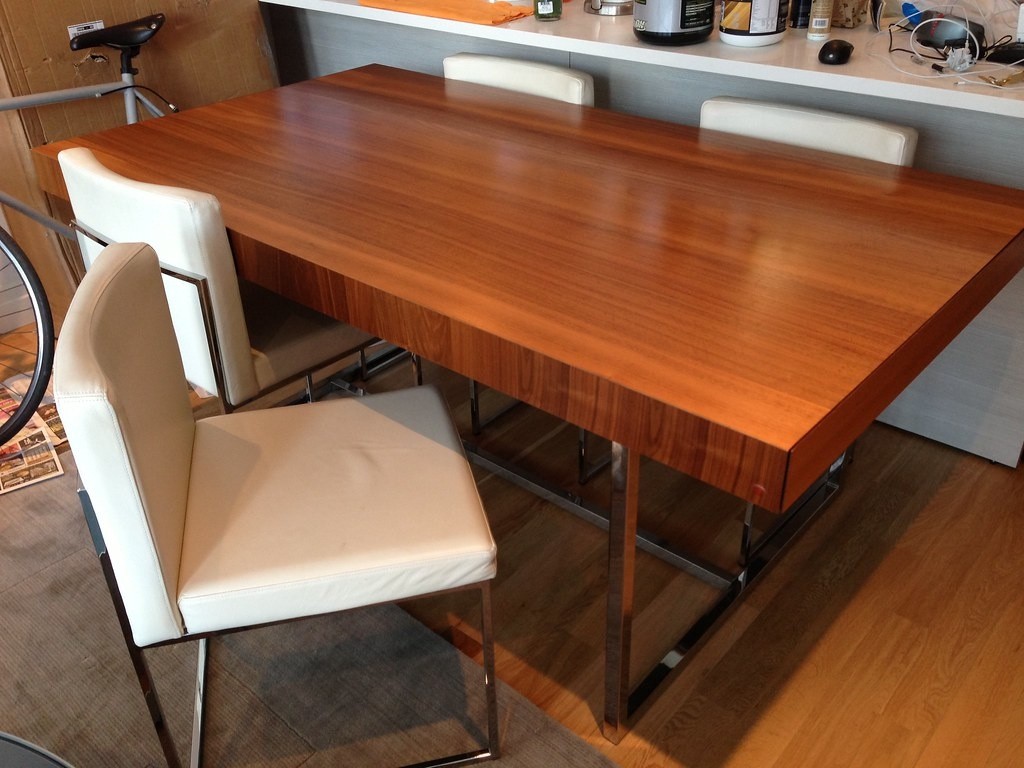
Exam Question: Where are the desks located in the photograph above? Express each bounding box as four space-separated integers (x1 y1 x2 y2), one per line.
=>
32 63 1024 746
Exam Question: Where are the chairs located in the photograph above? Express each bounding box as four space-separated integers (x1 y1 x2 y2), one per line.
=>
390 54 596 437
56 145 424 411
55 243 501 768
602 90 919 564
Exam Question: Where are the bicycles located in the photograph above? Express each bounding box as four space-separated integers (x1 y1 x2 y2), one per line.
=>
0 14 180 445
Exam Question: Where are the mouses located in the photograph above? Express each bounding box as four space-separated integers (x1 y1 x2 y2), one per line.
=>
818 39 855 65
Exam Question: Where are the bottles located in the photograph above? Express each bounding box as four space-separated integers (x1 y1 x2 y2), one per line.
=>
532 0 835 47
